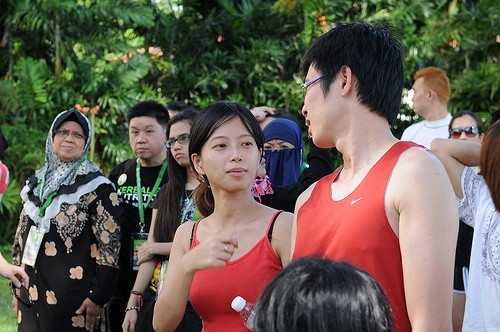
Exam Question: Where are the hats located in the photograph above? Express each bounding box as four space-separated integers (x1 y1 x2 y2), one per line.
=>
52 110 89 141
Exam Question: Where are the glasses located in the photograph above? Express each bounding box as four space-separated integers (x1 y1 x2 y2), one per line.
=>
54 129 87 140
9 281 34 312
301 69 341 99
450 126 481 140
166 134 191 148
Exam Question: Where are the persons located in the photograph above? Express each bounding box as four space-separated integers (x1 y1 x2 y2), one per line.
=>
0 130 30 291
247 105 336 211
400 67 500 332
153 100 293 332
107 98 205 332
12 106 121 332
257 254 395 332
291 22 458 332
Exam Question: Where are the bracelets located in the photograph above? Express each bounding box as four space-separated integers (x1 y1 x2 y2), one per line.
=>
125 307 138 312
130 290 143 310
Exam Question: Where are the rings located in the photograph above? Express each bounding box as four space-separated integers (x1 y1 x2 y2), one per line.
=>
224 245 228 252
96 316 100 319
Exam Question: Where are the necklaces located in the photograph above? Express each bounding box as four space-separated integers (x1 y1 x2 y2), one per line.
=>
183 184 195 205
